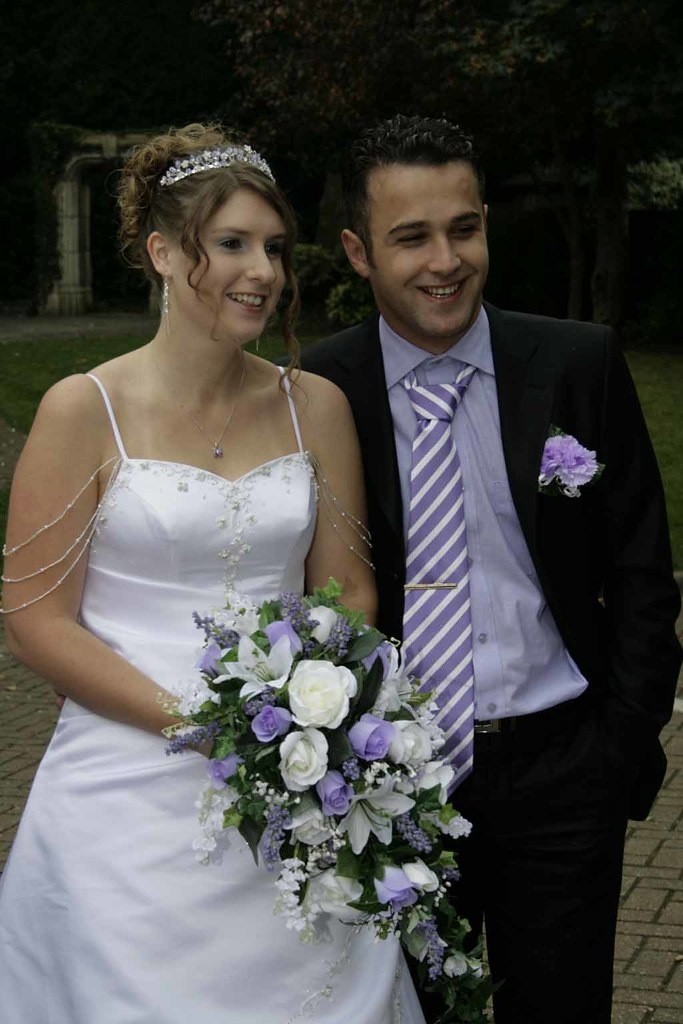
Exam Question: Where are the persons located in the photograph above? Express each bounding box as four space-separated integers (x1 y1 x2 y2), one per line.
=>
0 116 429 1024
278 107 683 1022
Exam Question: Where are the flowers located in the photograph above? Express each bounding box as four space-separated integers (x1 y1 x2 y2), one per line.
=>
156 576 500 1023
539 427 608 499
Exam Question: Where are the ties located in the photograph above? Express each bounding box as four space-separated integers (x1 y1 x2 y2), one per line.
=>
397 364 477 800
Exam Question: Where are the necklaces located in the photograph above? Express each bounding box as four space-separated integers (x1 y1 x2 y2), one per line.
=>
145 312 248 459
